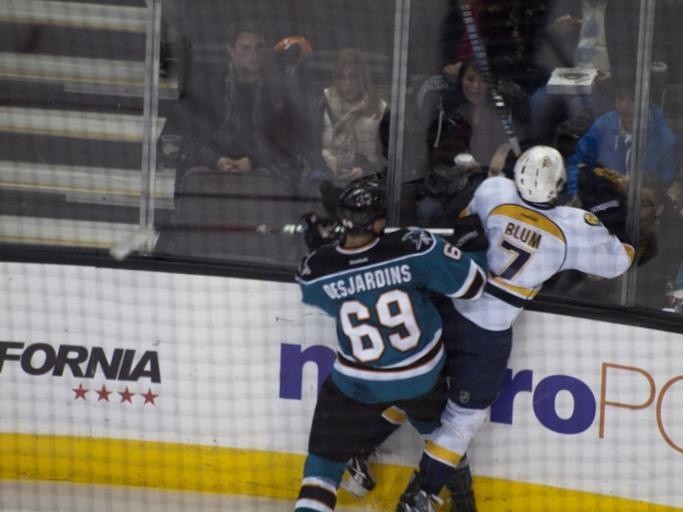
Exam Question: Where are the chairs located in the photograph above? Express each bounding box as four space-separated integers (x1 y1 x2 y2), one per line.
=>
152 0 682 316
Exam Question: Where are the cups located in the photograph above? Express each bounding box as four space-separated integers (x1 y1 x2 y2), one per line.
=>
162 133 183 162
453 153 476 170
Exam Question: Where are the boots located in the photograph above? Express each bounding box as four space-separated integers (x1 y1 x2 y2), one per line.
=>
447 467 477 512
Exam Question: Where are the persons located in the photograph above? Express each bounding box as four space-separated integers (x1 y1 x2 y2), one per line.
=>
344 145 635 512
293 178 488 512
153 1 682 307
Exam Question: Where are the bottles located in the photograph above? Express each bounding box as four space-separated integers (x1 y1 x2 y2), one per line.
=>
664 274 676 307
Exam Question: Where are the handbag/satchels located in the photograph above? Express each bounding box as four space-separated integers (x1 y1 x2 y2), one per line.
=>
424 164 478 195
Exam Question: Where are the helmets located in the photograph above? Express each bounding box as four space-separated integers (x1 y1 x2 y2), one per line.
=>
515 145 567 203
335 181 388 235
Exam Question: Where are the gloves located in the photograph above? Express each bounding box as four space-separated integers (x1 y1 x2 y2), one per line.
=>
579 177 626 234
303 212 338 251
502 149 517 179
456 214 489 251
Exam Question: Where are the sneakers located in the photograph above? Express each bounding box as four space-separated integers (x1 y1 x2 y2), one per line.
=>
399 484 443 512
345 456 375 491
